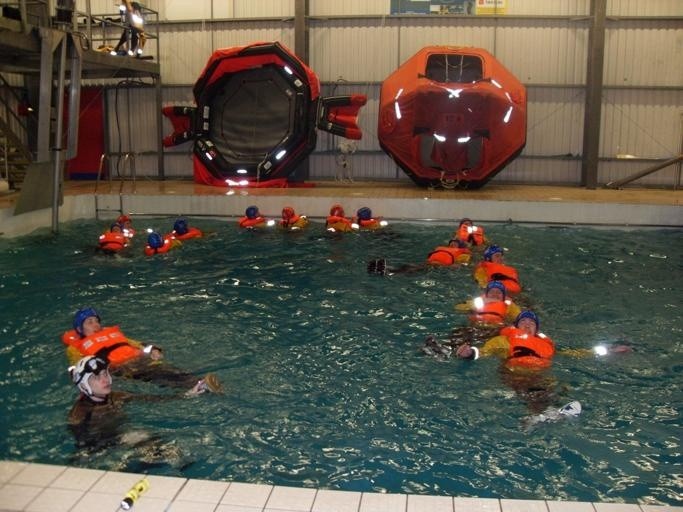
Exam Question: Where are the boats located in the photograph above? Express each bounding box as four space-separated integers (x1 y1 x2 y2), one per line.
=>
157 38 367 188
373 42 530 192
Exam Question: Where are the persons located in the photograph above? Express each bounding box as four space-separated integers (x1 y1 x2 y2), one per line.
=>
110 0 147 56
67 355 205 473
239 205 276 235
317 205 357 242
62 308 225 394
419 281 522 361
367 238 472 278
76 215 202 260
276 207 309 248
357 207 396 243
453 218 509 261
473 243 546 316
457 311 633 425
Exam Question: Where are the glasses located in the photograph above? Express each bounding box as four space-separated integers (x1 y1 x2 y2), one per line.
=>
75 356 110 385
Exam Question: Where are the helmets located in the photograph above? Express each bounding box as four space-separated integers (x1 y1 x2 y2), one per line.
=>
330 207 345 218
148 232 164 248
110 215 131 233
73 355 113 396
282 207 294 220
515 311 539 332
174 218 188 234
73 308 100 337
449 219 503 262
246 206 258 219
486 281 505 301
357 207 371 219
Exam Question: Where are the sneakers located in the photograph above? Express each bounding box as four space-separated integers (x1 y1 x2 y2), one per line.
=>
367 259 386 276
422 337 452 363
205 375 224 393
